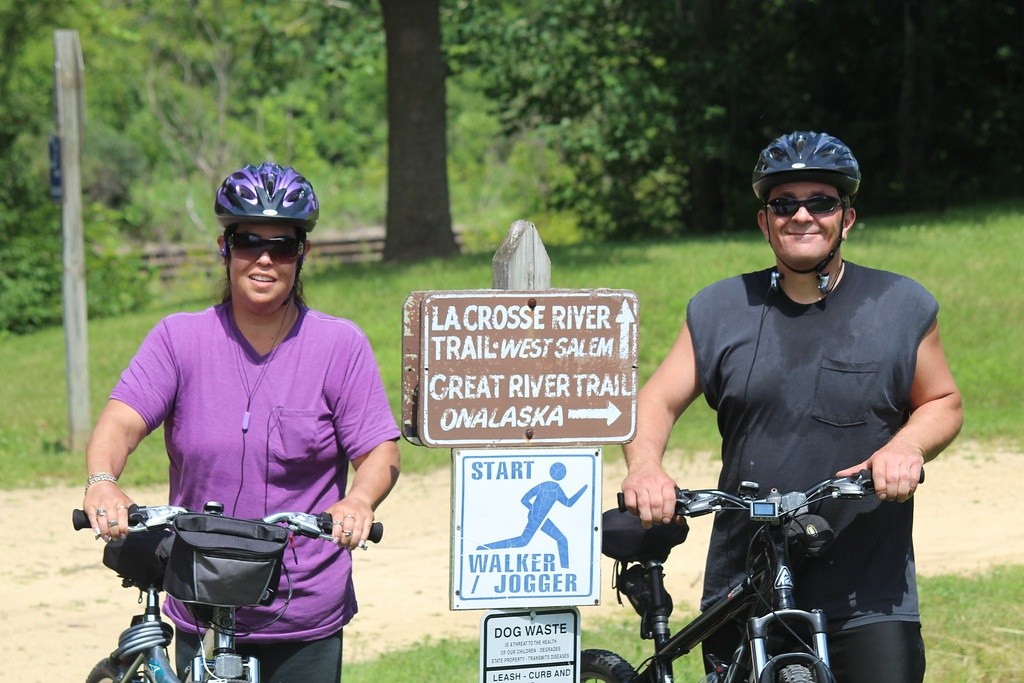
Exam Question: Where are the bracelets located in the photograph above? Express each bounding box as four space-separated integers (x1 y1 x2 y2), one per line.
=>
84 473 118 495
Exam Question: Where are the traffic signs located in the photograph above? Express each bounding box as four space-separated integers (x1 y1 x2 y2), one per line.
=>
413 288 642 449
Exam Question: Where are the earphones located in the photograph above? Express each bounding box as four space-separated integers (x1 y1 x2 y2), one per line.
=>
220 240 227 256
300 250 306 264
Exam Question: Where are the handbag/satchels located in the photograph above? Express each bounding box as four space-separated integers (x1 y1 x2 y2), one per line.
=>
164 513 289 607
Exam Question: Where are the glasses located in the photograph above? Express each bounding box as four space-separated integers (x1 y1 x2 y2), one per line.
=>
226 232 304 261
764 194 845 217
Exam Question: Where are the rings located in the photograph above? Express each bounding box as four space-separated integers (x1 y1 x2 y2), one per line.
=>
96 508 108 516
343 514 355 520
342 530 353 536
330 521 344 527
117 506 128 510
107 520 117 527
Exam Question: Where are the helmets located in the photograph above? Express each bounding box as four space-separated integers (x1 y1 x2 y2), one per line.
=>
213 162 319 233
751 131 861 200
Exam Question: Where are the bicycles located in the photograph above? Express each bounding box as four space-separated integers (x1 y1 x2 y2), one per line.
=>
70 501 385 683
578 467 927 683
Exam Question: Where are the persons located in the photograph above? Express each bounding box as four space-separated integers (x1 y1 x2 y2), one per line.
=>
80 161 404 683
619 129 967 683
